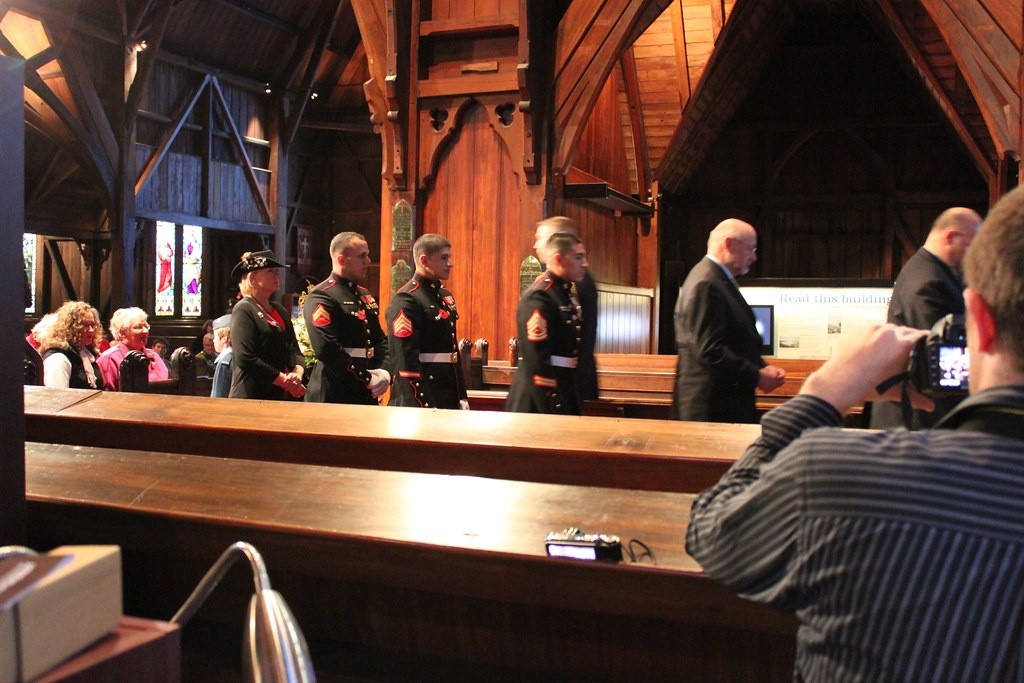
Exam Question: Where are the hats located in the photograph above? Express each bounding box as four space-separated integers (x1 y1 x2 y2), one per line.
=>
212 314 232 331
231 249 291 281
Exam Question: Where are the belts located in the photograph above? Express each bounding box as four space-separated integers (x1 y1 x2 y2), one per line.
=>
550 355 579 368
419 351 460 364
344 347 374 360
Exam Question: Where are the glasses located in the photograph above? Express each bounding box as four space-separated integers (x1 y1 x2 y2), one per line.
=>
731 236 757 255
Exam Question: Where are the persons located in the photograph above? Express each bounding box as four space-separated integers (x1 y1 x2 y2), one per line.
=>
211 314 233 399
25 301 110 389
195 334 217 376
232 250 307 402
385 234 469 409
97 306 168 392
303 232 393 406
686 181 1024 683
871 207 983 431
670 218 783 424
505 216 599 416
152 337 172 379
192 319 213 353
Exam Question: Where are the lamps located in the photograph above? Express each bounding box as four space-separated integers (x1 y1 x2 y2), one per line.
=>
168 539 320 682
264 83 272 93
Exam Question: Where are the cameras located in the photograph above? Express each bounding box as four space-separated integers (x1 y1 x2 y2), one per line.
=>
912 313 970 399
545 527 622 563
